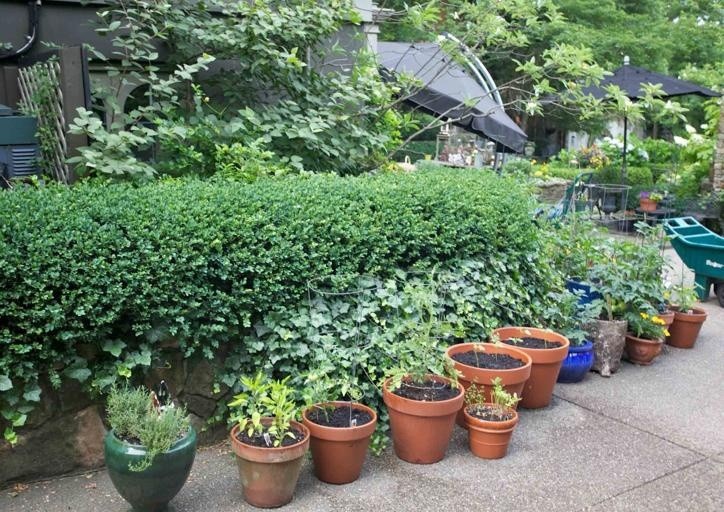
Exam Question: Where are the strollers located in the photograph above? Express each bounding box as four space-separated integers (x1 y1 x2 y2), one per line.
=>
529 171 592 233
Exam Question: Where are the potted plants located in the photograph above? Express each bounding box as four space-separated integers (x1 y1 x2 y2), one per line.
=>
103 375 197 512
555 296 606 384
445 342 532 430
464 375 523 460
626 220 708 365
301 359 378 484
565 220 605 307
227 369 310 508
381 278 465 463
490 326 570 411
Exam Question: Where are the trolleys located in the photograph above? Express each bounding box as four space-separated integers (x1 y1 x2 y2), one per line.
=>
662 216 723 307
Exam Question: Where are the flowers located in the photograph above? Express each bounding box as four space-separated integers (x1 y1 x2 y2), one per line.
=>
640 192 663 202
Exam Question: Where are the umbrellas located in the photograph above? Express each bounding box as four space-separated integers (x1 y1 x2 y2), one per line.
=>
535 63 724 192
374 36 527 159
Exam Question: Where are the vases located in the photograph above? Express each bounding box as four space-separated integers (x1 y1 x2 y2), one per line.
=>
640 199 657 210
